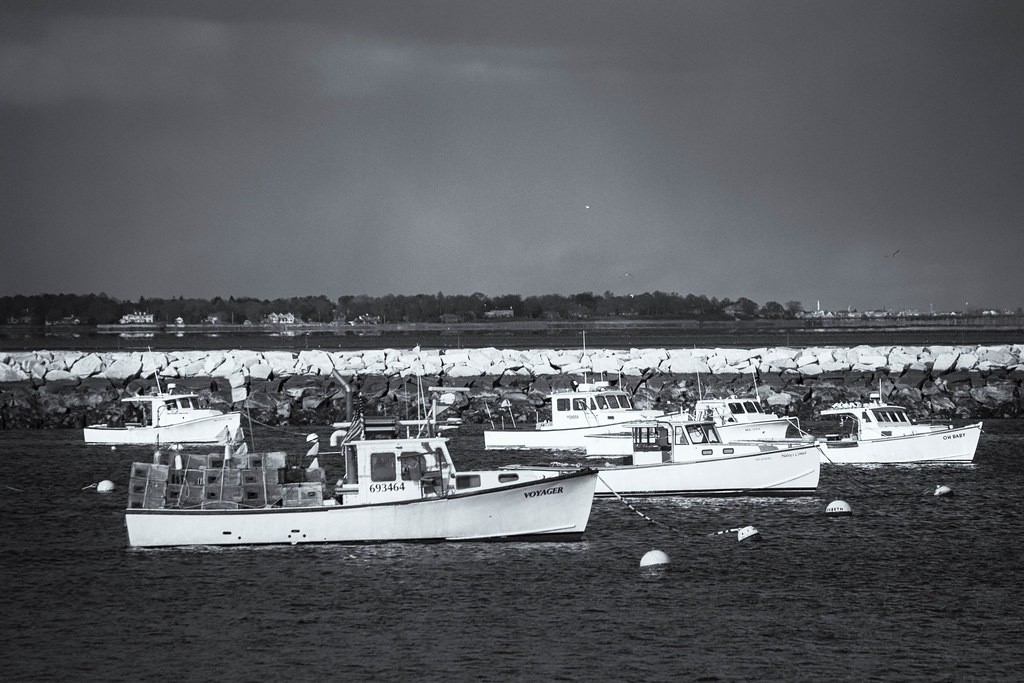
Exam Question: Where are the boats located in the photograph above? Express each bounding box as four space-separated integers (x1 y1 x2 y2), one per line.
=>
124 345 599 548
82 346 241 443
583 364 799 461
482 330 688 452
501 414 822 497
727 379 983 466
